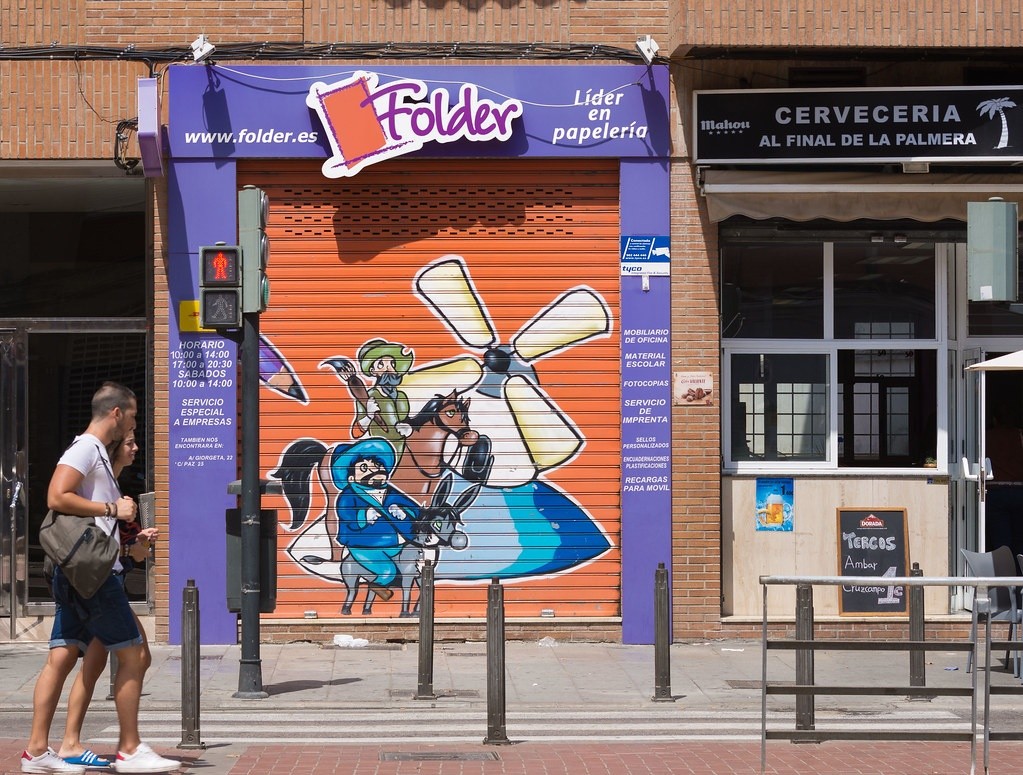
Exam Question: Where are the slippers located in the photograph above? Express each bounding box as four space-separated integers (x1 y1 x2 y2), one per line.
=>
63 749 110 766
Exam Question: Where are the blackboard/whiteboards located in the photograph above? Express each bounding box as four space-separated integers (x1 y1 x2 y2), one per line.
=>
836 508 911 616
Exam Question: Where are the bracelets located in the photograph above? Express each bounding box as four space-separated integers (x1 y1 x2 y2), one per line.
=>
123 543 130 558
104 502 118 521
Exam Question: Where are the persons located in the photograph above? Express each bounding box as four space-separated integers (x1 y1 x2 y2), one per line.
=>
846 411 936 467
731 432 766 462
21 383 181 775
985 400 1023 607
57 429 160 775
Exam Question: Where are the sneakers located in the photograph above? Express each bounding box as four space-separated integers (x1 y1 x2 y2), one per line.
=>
114 743 182 773
19 746 86 774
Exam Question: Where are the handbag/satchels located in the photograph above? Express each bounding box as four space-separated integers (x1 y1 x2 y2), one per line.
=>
40 508 118 595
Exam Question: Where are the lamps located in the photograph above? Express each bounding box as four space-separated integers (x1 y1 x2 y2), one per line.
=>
636 35 661 66
191 36 217 63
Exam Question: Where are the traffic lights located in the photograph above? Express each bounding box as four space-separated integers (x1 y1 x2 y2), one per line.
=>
200 242 243 328
238 184 269 313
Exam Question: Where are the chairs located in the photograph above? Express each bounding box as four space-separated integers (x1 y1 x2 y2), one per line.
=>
956 548 1023 684
